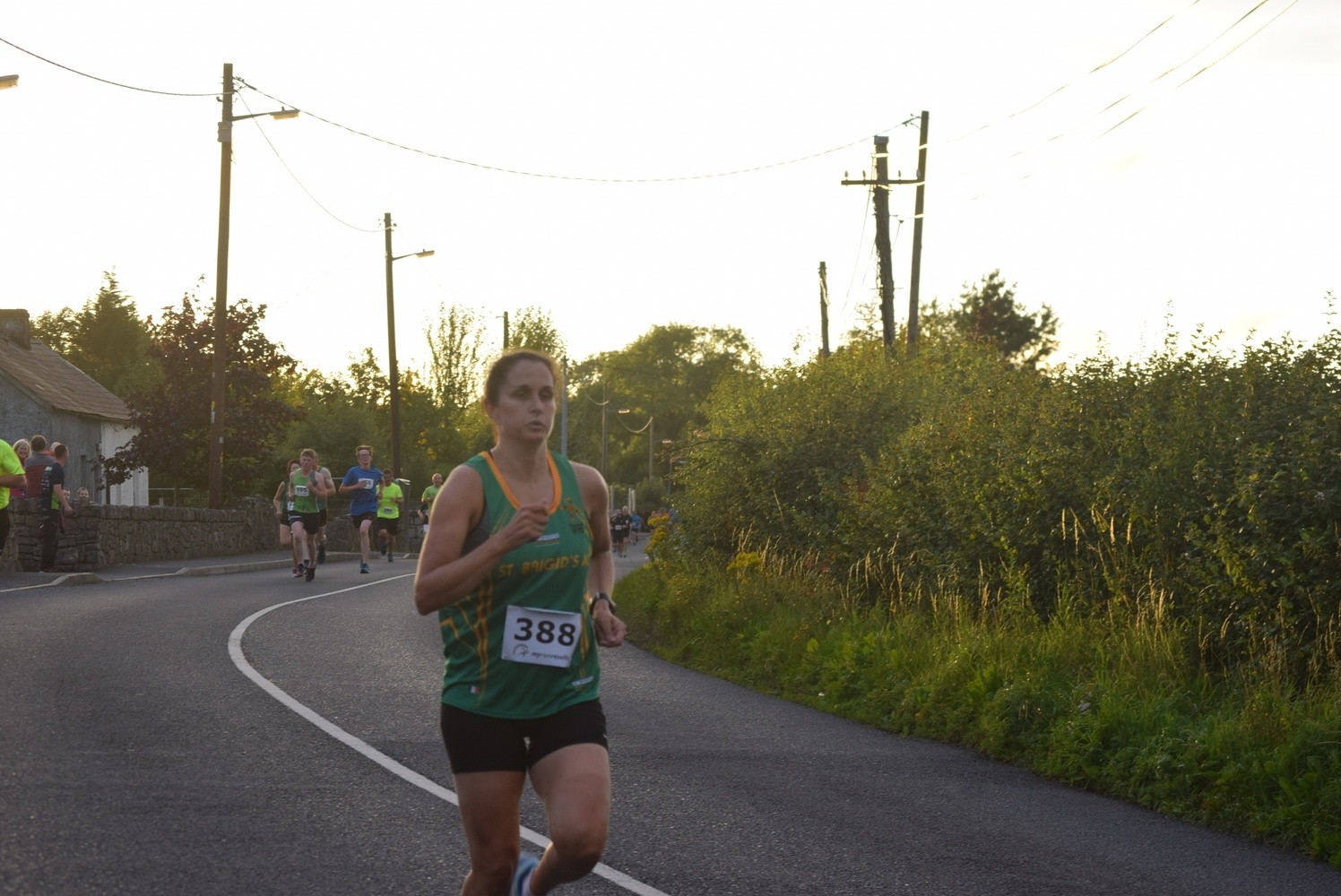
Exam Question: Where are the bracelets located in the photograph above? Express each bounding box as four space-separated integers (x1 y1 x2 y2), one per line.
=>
395 499 398 503
379 491 383 495
586 591 618 615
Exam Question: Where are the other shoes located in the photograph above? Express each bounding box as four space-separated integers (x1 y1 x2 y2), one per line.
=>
298 563 305 575
293 568 300 577
305 565 316 582
304 560 310 566
510 851 541 896
388 554 393 562
317 547 326 564
382 545 386 555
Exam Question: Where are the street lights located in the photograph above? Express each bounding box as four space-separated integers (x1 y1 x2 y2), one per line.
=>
648 438 673 483
208 105 301 508
386 248 435 478
601 408 631 477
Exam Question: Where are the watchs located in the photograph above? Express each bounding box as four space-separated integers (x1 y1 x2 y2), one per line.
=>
427 497 430 502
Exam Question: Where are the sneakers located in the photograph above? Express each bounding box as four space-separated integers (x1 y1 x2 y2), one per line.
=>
360 563 370 573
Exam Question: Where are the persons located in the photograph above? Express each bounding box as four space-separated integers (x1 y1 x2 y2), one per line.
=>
0 434 101 573
287 448 328 582
375 468 405 562
606 504 679 559
413 352 627 896
309 452 336 565
338 445 384 573
272 459 302 578
